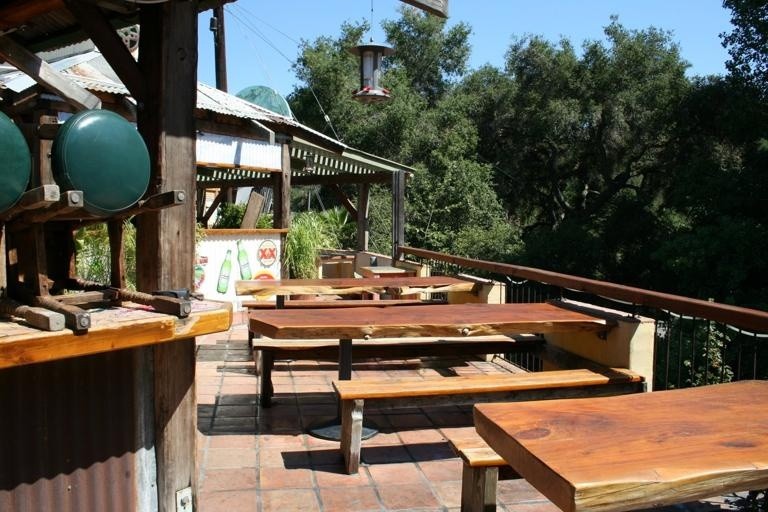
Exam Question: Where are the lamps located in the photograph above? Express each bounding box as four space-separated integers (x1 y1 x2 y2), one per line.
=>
351 0 393 103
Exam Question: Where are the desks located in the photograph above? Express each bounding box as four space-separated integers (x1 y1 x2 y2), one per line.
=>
235 276 476 310
248 303 606 442
359 265 406 300
474 380 768 512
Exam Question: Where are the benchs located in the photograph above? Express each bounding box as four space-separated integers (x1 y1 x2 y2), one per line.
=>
440 427 510 512
331 367 645 475
242 300 448 347
251 334 547 409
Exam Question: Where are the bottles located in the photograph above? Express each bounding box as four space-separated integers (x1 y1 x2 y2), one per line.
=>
216 249 231 294
236 239 252 280
